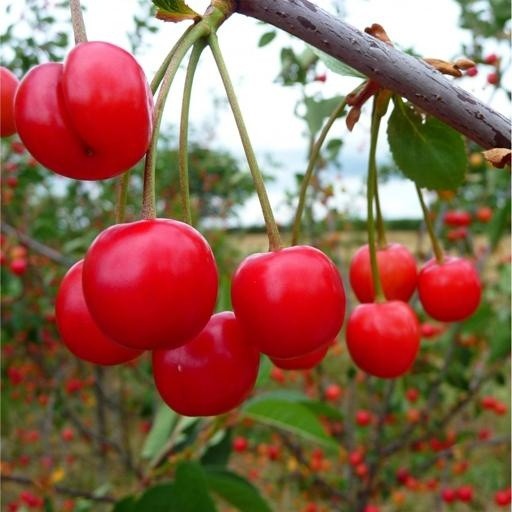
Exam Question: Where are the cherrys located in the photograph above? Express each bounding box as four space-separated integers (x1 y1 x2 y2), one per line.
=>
1 41 511 512
